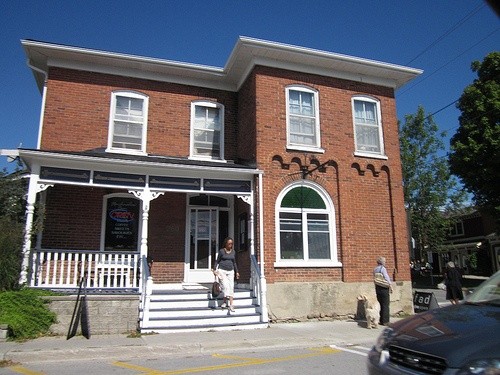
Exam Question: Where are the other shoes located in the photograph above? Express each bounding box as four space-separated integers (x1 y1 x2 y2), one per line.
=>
380 321 392 326
228 305 235 312
221 302 227 308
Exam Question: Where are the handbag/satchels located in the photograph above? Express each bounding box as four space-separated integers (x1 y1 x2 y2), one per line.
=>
373 272 390 288
212 282 220 297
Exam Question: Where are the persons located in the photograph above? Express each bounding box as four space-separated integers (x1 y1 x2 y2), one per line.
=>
444 261 464 304
373 257 393 326
213 237 239 312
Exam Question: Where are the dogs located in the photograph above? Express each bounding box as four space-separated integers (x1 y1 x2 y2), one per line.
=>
365 297 380 329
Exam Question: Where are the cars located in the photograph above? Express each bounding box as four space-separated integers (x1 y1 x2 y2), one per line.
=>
366 271 500 375
